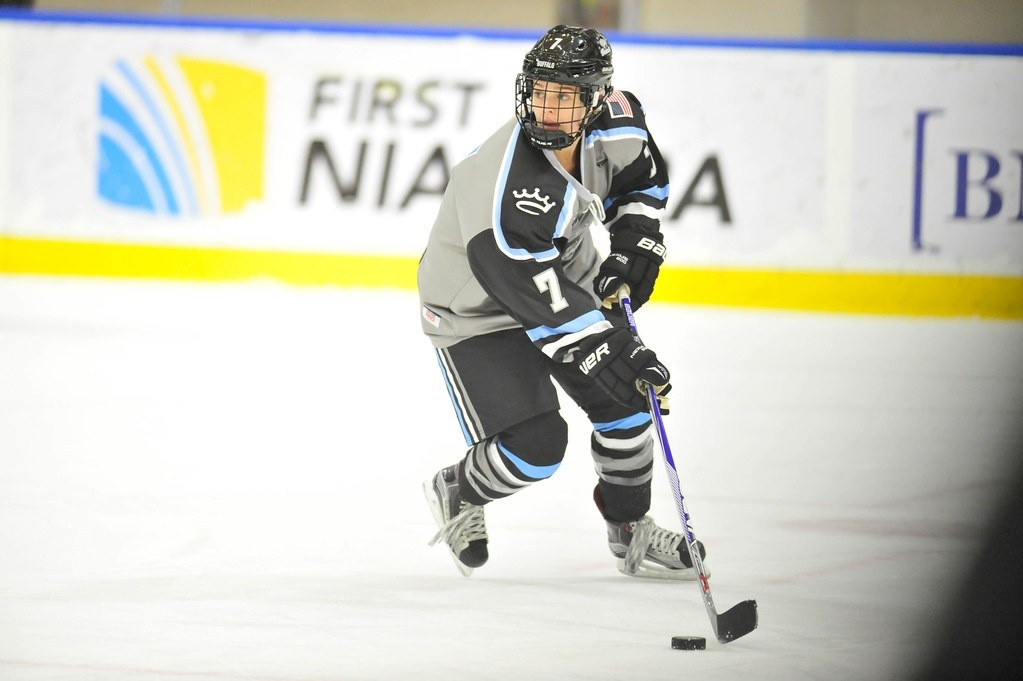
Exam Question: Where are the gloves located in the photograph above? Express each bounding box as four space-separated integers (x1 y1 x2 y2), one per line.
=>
574 327 672 414
593 228 665 316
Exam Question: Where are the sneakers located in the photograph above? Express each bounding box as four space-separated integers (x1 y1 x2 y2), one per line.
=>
594 482 711 581
423 463 489 577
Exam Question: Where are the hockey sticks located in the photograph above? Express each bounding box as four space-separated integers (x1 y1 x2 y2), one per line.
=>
615 282 760 646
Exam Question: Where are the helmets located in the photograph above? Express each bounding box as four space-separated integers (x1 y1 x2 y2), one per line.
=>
513 25 613 150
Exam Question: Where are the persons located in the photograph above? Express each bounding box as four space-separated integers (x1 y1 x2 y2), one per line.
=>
415 24 706 579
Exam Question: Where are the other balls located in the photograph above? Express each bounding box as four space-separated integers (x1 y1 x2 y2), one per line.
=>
670 634 707 651
